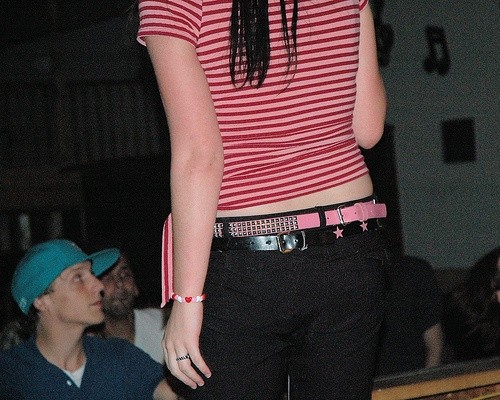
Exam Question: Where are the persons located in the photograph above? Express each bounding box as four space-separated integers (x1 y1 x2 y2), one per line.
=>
135 0 389 400
78 253 166 370
0 238 181 400
376 249 500 376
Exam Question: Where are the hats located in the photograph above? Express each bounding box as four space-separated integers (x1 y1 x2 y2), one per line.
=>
12 239 119 315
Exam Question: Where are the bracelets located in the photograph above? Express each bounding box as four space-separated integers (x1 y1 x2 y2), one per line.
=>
170 292 206 304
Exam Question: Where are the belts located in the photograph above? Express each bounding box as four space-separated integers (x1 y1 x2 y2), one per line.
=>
213 198 387 237
211 217 381 252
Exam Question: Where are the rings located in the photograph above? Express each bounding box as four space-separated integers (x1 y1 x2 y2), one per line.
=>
175 353 190 361
